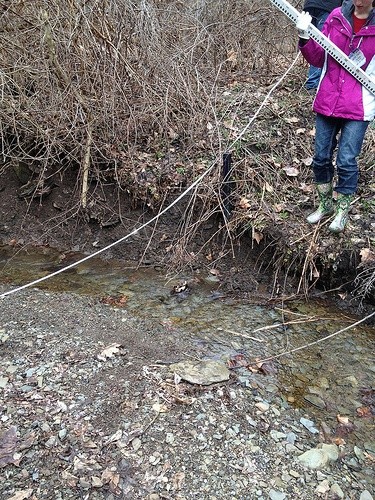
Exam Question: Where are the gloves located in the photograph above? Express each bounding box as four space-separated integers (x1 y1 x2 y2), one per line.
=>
296 11 313 40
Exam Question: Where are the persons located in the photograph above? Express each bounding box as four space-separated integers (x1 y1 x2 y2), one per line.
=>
295 0 375 232
301 0 341 91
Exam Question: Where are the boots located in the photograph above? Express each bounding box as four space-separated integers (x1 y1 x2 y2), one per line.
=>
307 181 333 224
329 191 351 232
